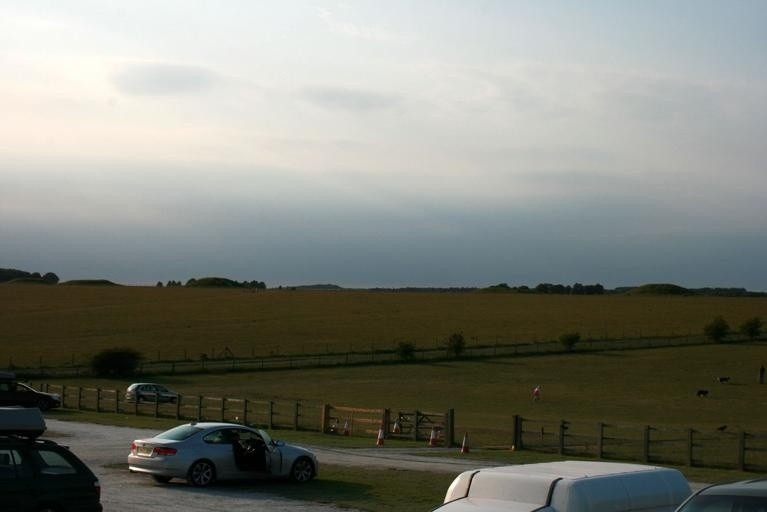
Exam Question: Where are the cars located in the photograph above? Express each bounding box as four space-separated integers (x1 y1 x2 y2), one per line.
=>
0 382 61 412
127 422 318 488
677 478 767 512
435 461 689 512
125 383 182 404
0 438 104 512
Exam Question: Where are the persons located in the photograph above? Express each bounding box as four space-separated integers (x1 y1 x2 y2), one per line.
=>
533 385 544 402
233 432 248 453
758 364 765 384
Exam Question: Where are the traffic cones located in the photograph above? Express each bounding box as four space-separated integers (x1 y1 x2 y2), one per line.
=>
393 423 400 432
343 421 349 435
376 426 472 454
